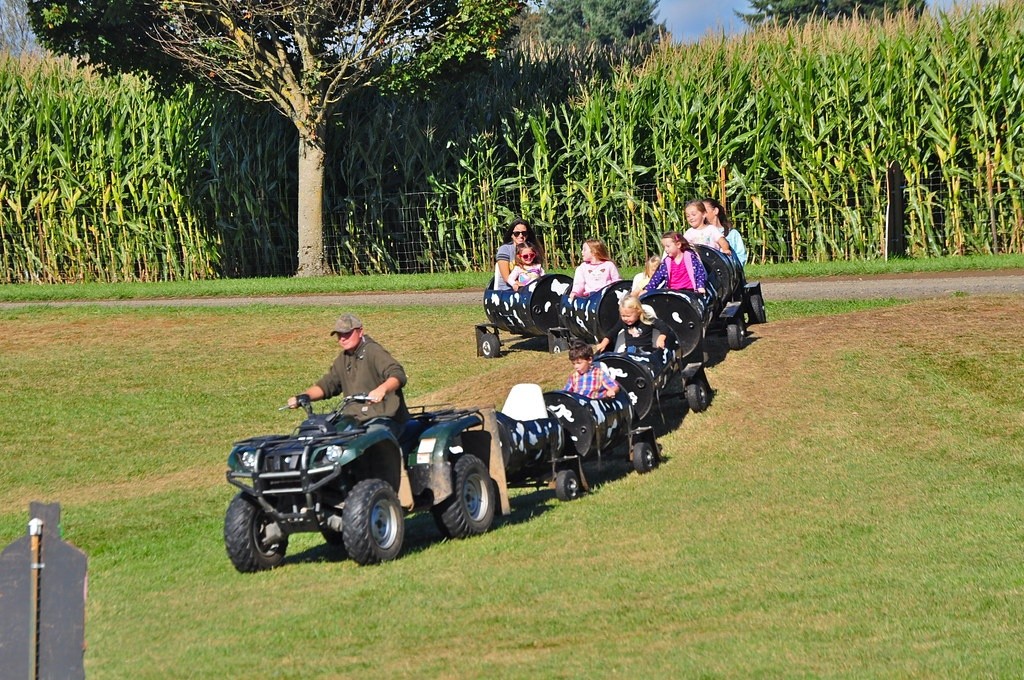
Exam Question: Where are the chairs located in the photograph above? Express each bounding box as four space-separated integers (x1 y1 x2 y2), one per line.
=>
501 383 548 421
593 362 609 376
493 262 511 291
614 304 659 353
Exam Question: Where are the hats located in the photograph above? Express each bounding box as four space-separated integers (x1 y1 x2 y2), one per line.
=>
330 313 362 336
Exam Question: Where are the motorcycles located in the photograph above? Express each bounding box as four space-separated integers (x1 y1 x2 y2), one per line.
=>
223 393 498 574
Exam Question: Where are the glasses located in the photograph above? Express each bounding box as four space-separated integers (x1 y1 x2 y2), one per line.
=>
512 231 528 237
518 252 535 260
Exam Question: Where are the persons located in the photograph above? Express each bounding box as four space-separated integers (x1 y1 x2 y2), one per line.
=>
683 198 746 268
493 219 546 291
287 314 421 464
569 238 619 301
632 230 706 297
564 343 620 399
596 297 671 352
